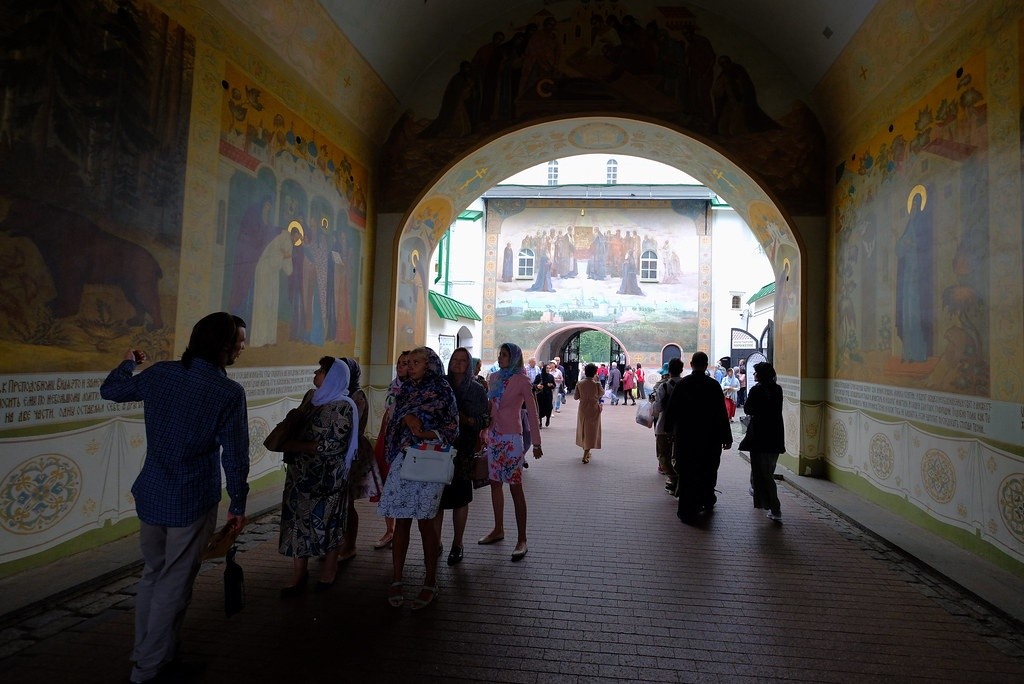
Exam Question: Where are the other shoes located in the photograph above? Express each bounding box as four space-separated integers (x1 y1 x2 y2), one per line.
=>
657 467 664 474
337 547 356 561
616 399 619 405
555 409 560 413
664 484 675 494
546 418 550 426
582 459 589 463
374 533 393 548
511 544 528 556
320 563 337 583
622 403 627 405
283 570 309 591
478 531 504 544
632 402 636 405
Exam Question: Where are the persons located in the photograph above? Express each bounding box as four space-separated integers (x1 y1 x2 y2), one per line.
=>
100 312 250 684
737 362 787 520
565 365 577 393
649 352 733 519
526 356 565 428
278 343 544 609
713 359 747 422
573 364 605 463
597 362 647 406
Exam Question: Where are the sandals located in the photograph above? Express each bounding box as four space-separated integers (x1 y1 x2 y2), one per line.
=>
410 580 439 609
388 580 403 608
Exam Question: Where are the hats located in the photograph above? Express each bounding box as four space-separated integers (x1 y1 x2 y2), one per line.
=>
659 363 669 375
754 362 776 382
550 360 557 365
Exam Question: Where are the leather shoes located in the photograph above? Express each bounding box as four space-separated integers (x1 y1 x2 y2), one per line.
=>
449 545 463 560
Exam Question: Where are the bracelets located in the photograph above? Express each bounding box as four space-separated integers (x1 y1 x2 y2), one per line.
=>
534 446 541 449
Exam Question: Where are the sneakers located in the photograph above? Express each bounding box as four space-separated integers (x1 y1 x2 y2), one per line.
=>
767 512 781 520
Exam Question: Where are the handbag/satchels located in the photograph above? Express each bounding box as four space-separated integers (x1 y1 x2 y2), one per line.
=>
723 388 727 396
632 382 636 389
400 429 456 484
262 391 319 452
603 389 612 399
472 445 492 489
636 401 653 428
